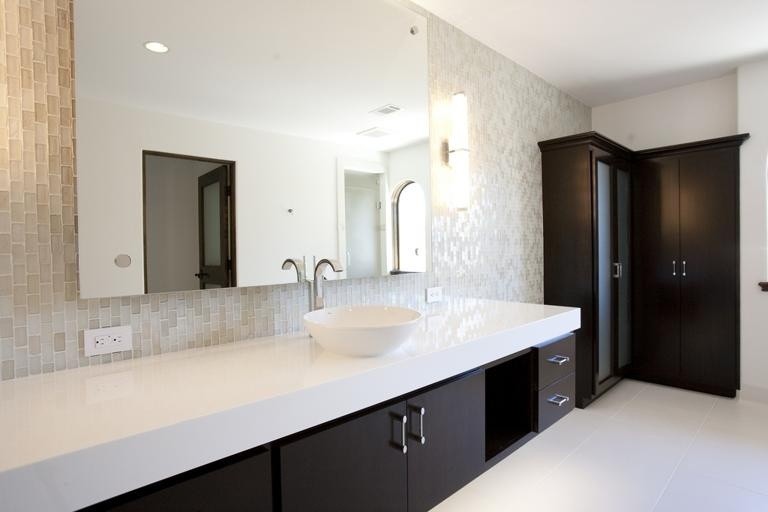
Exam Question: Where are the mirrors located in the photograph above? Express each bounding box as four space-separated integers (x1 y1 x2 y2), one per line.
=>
72 0 431 300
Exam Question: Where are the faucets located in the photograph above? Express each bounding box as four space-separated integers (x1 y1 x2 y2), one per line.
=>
282 257 305 283
304 255 343 338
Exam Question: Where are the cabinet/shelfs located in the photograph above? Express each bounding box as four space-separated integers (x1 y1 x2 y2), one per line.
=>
103 444 277 512
277 369 485 512
537 130 630 410
534 333 576 434
630 133 751 399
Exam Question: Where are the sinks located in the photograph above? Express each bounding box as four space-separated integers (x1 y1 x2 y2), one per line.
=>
303 305 423 359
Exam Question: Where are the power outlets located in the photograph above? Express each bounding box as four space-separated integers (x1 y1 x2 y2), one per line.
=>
426 286 443 303
83 326 132 357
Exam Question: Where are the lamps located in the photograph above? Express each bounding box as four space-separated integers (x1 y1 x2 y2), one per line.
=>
449 92 471 171
